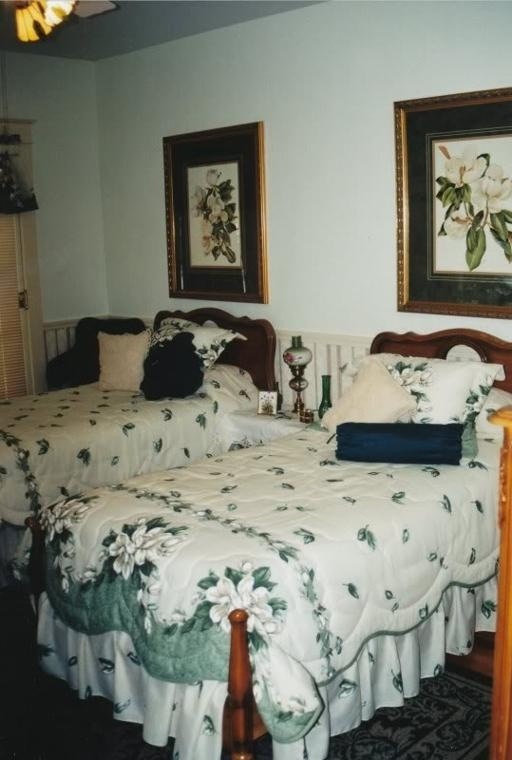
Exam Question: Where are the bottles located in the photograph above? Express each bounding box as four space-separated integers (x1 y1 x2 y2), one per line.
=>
317 374 333 421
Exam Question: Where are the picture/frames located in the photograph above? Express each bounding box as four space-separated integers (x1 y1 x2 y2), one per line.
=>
392 87 512 321
162 121 270 307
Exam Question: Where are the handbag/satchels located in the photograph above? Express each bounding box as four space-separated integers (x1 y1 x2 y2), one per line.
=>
0 157 38 214
335 422 463 465
140 332 203 400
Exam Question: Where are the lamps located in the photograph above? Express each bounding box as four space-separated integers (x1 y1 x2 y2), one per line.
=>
3 0 84 47
